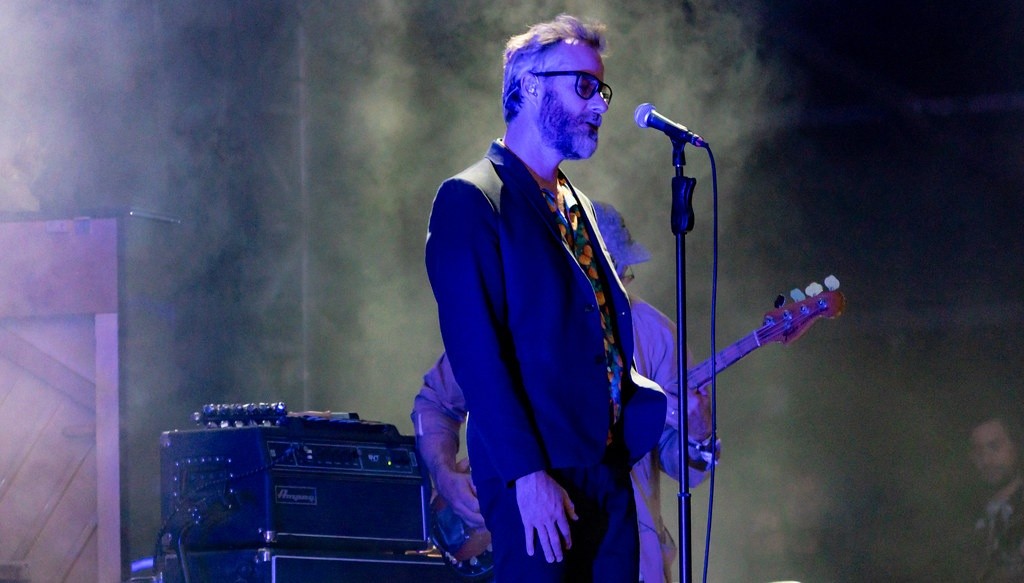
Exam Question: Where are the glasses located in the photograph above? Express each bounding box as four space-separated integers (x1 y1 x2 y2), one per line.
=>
534 70 613 106
620 265 635 285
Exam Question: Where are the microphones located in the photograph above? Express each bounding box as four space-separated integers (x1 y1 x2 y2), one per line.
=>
633 102 704 148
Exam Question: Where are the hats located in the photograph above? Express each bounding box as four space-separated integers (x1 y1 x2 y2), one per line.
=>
592 200 653 265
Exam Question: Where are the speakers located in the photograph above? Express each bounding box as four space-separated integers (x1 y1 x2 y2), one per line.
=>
161 549 457 583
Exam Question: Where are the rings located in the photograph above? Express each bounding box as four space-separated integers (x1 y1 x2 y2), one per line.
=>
671 409 675 415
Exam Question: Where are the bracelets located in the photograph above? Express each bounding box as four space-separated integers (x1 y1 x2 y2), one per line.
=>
689 455 721 473
689 437 717 453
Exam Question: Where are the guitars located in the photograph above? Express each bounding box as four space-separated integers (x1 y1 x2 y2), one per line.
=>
429 272 849 569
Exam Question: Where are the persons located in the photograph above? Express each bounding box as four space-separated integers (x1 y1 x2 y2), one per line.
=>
413 201 722 582
968 413 1024 582
427 15 667 583
762 464 849 583
